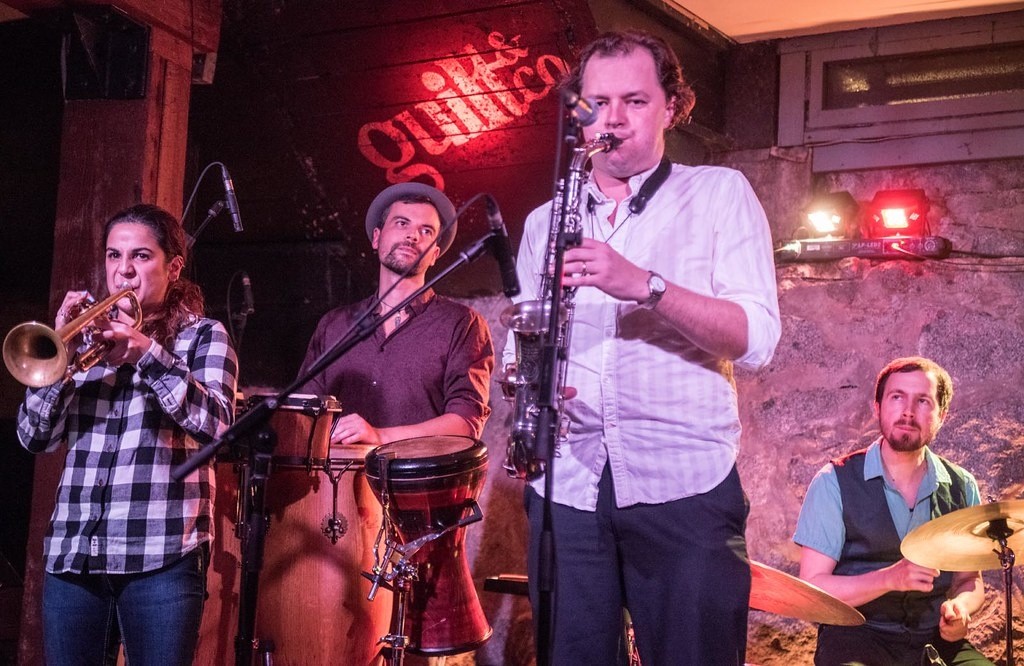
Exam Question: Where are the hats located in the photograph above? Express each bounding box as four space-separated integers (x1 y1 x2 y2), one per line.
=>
364 183 457 260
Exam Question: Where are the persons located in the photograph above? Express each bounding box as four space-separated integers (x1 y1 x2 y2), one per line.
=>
497 26 785 666
790 353 1004 666
287 180 496 458
16 204 241 666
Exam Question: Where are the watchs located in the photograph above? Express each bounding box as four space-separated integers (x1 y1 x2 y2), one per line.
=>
636 269 668 311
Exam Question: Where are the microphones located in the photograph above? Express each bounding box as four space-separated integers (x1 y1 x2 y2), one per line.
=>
485 194 521 298
924 644 947 666
221 164 243 232
560 91 600 127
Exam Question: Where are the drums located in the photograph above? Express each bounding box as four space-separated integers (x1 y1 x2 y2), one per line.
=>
248 393 392 666
363 435 494 656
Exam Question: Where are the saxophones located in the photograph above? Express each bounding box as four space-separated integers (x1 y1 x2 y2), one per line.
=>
498 131 623 481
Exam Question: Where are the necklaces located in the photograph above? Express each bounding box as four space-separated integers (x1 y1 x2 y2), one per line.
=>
378 296 413 331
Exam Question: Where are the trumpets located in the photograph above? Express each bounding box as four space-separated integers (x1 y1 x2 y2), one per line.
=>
3 281 142 388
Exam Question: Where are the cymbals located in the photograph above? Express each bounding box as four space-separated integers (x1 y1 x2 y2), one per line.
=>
749 558 867 626
900 499 1024 572
